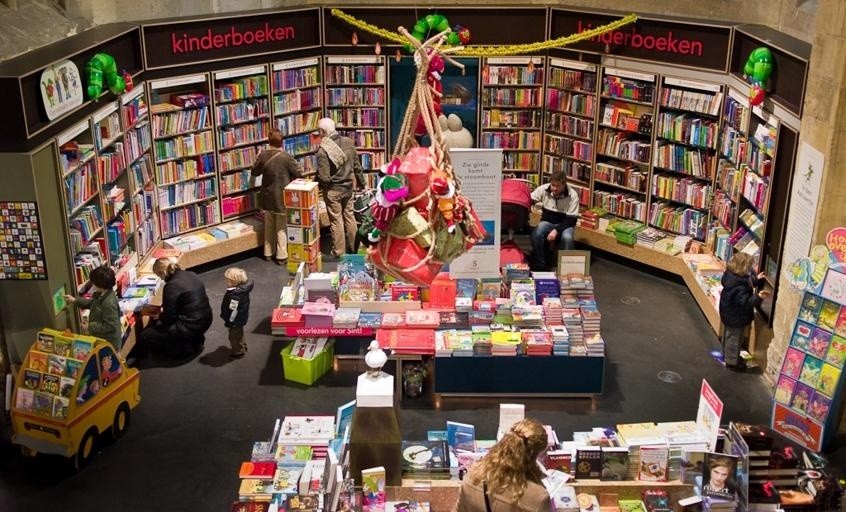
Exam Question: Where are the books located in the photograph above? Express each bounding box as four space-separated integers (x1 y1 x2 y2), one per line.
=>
238 404 844 512
57 67 781 371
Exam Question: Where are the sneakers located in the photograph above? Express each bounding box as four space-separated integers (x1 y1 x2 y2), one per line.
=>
229 345 247 358
722 354 747 370
266 255 287 265
322 254 343 262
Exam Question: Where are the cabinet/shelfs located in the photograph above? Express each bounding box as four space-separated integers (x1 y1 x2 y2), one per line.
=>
0 26 160 365
706 22 811 327
549 5 748 245
106 4 322 239
321 3 549 191
